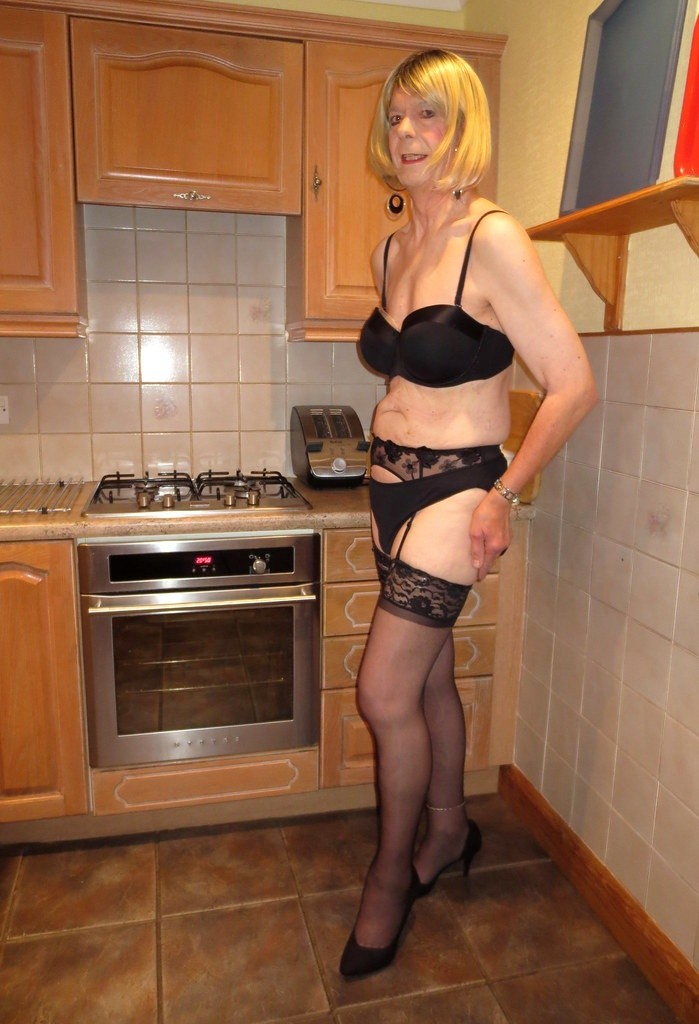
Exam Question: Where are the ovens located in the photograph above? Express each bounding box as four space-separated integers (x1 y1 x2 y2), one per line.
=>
74 529 324 770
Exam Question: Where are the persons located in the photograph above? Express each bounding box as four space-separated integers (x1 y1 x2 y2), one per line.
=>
340 49 600 974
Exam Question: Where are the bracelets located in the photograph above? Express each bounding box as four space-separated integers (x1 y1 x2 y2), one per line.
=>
424 801 466 811
493 480 517 501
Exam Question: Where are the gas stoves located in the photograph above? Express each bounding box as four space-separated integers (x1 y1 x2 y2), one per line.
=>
81 469 313 519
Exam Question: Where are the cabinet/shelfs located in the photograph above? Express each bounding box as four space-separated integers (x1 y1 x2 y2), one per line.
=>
318 527 501 790
0 0 508 342
0 539 90 845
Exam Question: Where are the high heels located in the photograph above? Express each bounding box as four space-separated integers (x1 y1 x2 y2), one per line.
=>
410 817 481 900
340 867 420 977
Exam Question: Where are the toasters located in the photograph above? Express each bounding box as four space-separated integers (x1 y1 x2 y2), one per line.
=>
291 405 369 489
